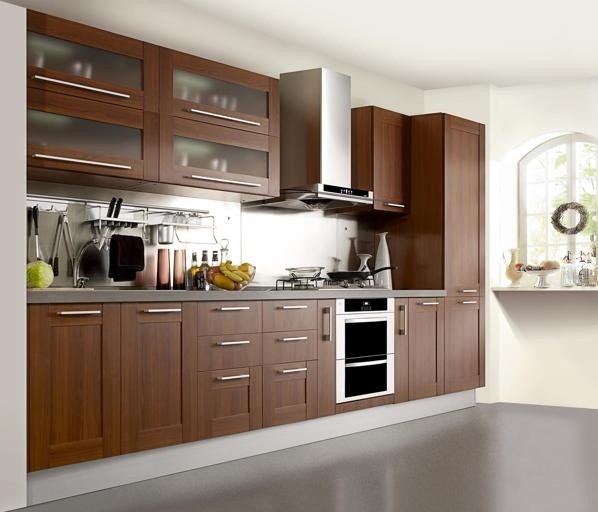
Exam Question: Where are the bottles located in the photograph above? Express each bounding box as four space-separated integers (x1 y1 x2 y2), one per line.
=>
199 250 210 290
220 248 229 265
212 251 219 266
188 252 201 289
560 251 575 288
579 259 598 286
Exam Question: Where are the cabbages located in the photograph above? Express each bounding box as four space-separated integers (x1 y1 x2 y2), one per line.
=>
27 261 53 288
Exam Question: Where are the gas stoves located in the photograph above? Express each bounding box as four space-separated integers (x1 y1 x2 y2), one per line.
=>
242 279 397 314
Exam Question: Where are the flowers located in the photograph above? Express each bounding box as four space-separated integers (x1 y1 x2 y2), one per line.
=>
551 202 588 236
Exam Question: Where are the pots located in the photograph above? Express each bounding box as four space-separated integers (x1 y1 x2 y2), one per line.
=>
326 265 397 282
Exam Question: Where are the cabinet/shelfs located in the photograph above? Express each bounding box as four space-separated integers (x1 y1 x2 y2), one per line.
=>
26 9 160 111
374 113 485 297
443 296 486 394
262 299 335 428
26 304 120 472
121 302 197 454
335 298 409 413
408 298 444 401
160 45 280 135
27 88 159 192
159 114 280 203
324 105 410 217
197 301 262 441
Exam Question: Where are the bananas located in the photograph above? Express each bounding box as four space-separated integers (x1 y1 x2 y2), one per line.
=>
219 260 250 282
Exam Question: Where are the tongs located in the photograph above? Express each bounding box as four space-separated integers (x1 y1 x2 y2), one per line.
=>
48 214 73 277
53 214 77 277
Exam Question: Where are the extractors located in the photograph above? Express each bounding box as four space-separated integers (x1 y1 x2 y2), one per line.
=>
239 67 374 211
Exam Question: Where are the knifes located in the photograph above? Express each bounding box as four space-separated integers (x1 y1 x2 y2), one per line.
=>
95 197 117 251
102 197 123 251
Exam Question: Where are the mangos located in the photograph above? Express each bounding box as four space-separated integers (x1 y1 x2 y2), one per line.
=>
213 273 235 290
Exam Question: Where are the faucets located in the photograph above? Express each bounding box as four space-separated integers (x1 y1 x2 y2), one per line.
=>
71 236 100 289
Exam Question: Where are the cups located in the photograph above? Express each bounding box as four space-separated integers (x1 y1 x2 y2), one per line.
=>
156 248 171 290
173 249 187 289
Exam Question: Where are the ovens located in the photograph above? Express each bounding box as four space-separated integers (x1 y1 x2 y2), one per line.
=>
336 311 395 404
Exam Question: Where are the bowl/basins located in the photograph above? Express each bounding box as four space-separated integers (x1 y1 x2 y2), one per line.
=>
284 266 325 282
206 264 256 292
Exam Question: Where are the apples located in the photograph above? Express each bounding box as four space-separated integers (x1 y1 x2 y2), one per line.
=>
234 282 242 290
238 263 254 277
208 268 219 282
